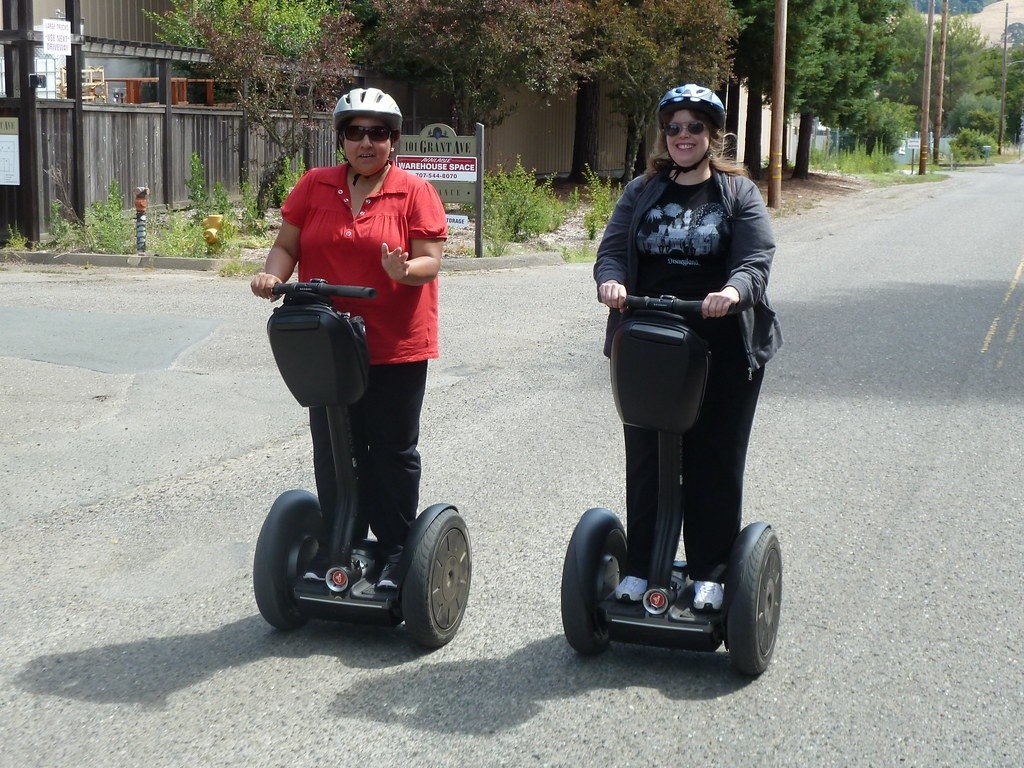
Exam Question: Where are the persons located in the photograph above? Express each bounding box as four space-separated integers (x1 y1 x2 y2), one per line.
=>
593 84 785 613
249 88 449 592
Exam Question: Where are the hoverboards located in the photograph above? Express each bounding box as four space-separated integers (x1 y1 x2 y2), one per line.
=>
253 278 473 651
561 296 785 682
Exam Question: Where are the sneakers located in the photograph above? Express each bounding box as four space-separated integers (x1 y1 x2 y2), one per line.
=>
377 563 399 590
694 580 724 610
616 576 648 602
303 559 330 581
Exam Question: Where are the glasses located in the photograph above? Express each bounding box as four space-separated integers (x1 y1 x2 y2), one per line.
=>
662 120 708 137
342 125 392 142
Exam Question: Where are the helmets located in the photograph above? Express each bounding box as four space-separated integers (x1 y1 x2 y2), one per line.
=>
657 83 726 131
333 88 401 131
